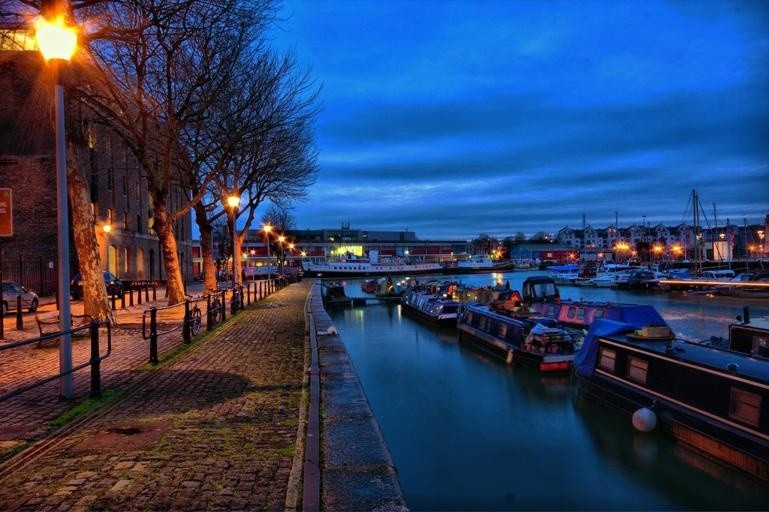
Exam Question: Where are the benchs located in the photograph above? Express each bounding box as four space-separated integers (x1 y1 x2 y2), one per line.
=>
35 310 98 349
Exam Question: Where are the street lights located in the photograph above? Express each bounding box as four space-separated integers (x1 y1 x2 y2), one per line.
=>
103 225 111 274
278 234 285 275
289 242 295 268
33 12 81 401
243 251 249 285
250 249 257 281
263 224 272 280
226 194 239 288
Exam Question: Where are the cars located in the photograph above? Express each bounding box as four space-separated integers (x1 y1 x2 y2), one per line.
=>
71 271 124 300
1 280 39 314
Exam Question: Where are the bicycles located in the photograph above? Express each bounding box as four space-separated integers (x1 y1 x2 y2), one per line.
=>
186 283 245 339
